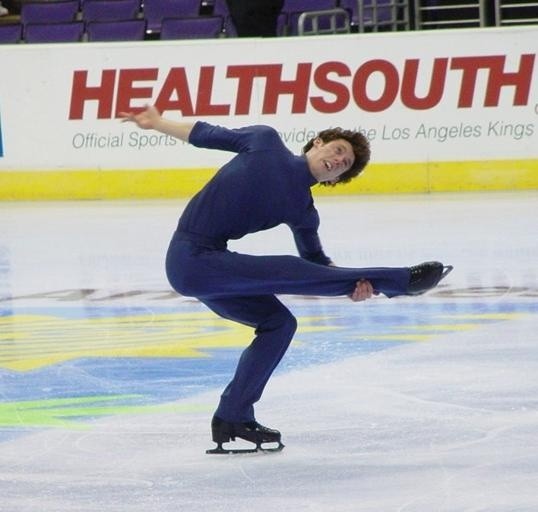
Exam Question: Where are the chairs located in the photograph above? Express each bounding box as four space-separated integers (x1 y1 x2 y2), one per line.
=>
1 0 400 43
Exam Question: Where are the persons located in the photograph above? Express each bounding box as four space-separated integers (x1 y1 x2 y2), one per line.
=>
118 101 453 454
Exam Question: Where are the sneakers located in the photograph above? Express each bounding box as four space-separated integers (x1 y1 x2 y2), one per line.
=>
212 414 281 441
411 261 443 294
